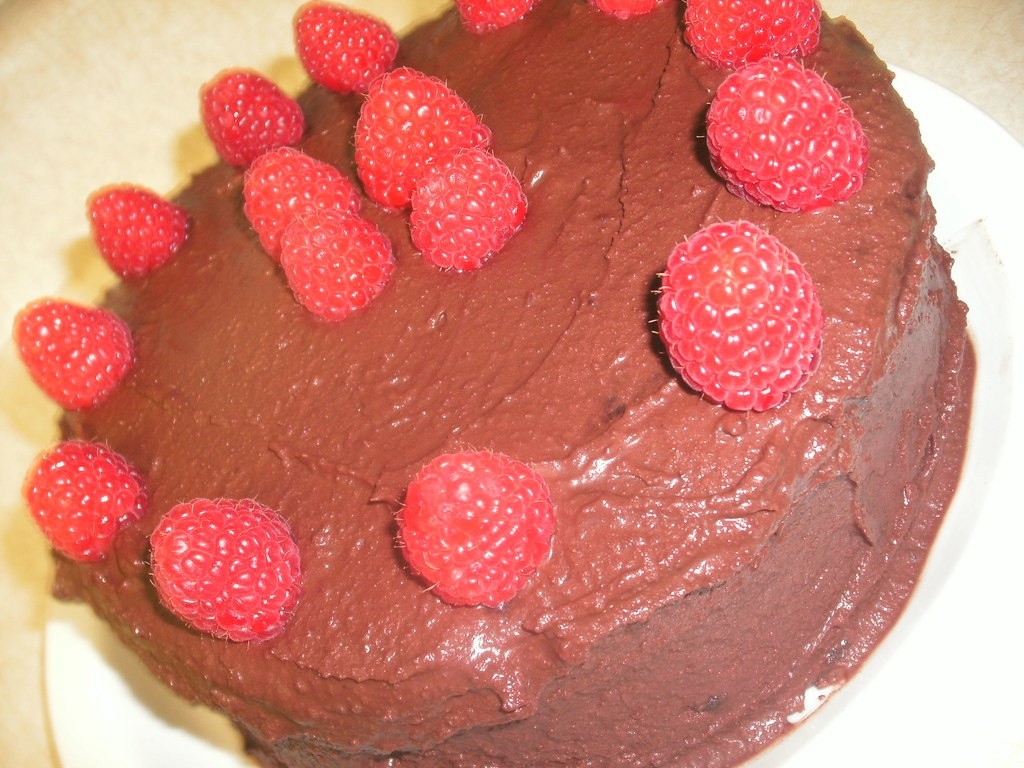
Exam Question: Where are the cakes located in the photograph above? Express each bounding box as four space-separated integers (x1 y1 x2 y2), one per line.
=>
14 0 976 768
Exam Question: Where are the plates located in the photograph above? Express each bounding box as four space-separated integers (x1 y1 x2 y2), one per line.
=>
42 65 1024 768
